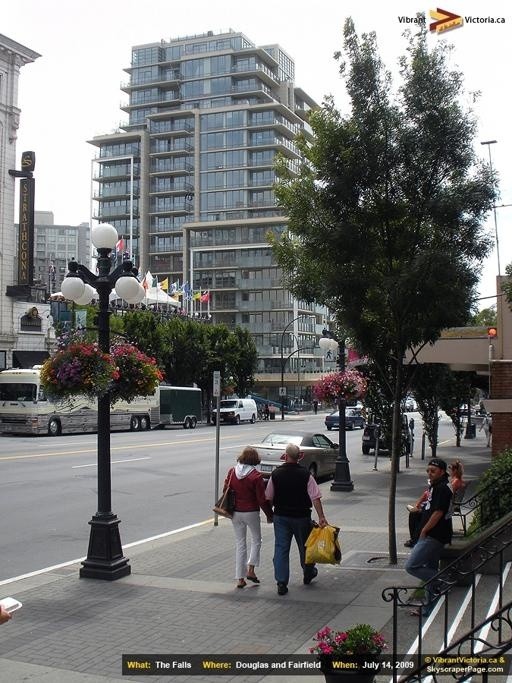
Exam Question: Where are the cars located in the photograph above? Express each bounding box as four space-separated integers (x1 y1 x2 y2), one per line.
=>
457 401 484 427
246 431 339 482
353 394 442 413
325 408 366 430
281 394 322 411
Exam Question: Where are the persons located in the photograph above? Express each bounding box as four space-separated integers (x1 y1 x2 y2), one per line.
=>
223 444 275 589
264 443 328 596
405 479 454 547
313 397 319 413
447 458 464 494
402 459 452 617
479 410 492 448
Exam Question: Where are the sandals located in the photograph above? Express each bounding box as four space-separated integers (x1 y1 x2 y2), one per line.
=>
246 575 260 583
237 581 247 588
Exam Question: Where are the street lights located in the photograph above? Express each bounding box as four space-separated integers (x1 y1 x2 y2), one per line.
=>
280 313 317 417
477 137 501 275
59 223 147 583
318 326 356 494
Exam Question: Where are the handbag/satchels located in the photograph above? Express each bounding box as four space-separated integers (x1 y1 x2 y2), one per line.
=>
212 467 236 520
304 523 343 565
489 423 492 433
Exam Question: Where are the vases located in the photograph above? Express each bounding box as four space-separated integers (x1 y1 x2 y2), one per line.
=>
322 656 379 682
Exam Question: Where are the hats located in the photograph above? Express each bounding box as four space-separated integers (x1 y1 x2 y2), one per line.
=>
429 457 450 477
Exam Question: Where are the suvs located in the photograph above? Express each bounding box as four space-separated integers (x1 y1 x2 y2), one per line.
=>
361 412 414 455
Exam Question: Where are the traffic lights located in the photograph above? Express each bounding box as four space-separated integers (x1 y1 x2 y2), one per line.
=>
487 326 498 337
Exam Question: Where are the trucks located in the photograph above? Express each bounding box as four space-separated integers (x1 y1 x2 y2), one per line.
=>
148 385 205 428
0 362 150 436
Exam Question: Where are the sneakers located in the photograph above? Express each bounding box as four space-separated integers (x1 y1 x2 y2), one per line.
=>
277 583 289 595
406 504 414 513
303 567 319 585
403 540 413 548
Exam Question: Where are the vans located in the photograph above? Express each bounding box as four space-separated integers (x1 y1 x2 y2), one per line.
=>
211 397 258 424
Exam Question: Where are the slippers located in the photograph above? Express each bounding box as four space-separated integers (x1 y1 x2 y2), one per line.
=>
409 609 428 617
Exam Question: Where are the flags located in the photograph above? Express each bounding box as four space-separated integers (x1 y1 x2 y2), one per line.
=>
137 269 210 303
108 236 136 265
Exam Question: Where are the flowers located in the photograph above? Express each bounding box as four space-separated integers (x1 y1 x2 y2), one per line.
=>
314 367 368 404
39 343 163 402
306 625 388 662
